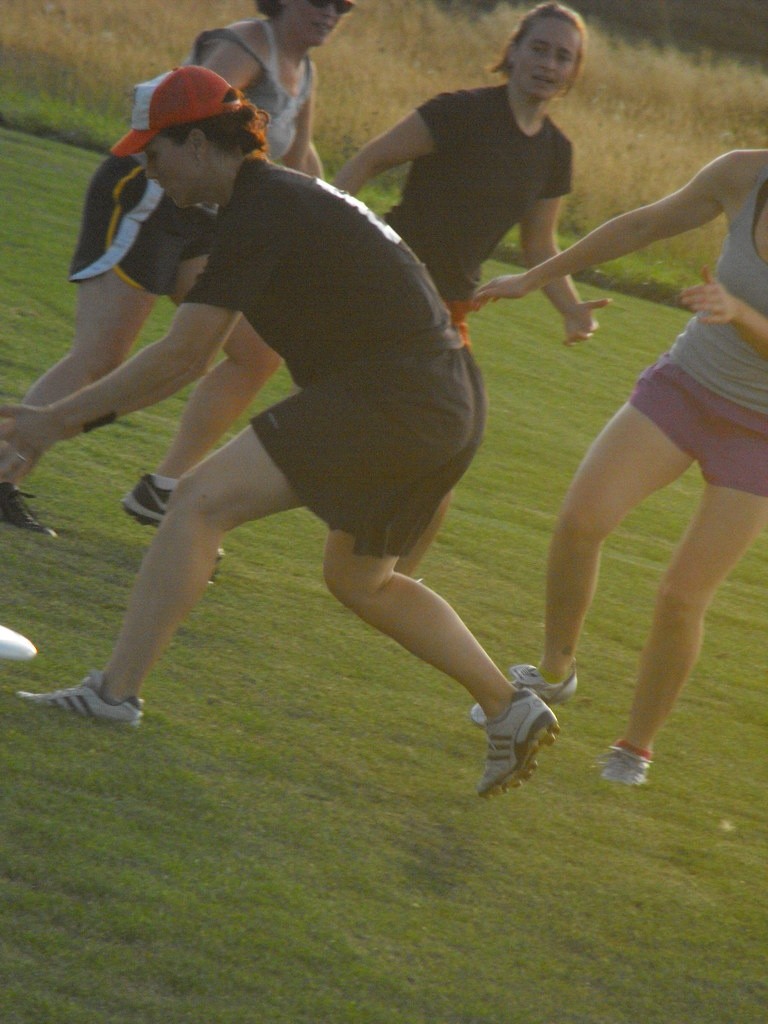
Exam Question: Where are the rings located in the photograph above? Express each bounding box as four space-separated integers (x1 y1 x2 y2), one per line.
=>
16 453 26 462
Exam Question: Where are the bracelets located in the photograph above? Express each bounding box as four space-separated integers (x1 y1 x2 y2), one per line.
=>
46 403 68 439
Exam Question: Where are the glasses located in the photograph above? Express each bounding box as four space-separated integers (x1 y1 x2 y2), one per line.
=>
304 0 355 15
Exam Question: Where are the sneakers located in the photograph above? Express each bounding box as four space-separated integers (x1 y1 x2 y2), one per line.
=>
0 482 57 538
120 472 225 561
477 690 561 799
470 664 576 729
602 739 654 787
17 668 143 729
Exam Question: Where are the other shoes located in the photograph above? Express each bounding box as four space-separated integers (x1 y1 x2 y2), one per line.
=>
0 623 36 660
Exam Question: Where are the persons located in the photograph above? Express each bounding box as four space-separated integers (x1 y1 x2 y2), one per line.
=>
0 66 562 798
334 3 612 577
0 1 353 559
470 149 768 788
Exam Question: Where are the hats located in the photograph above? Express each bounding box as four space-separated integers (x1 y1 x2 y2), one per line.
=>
110 65 252 156
